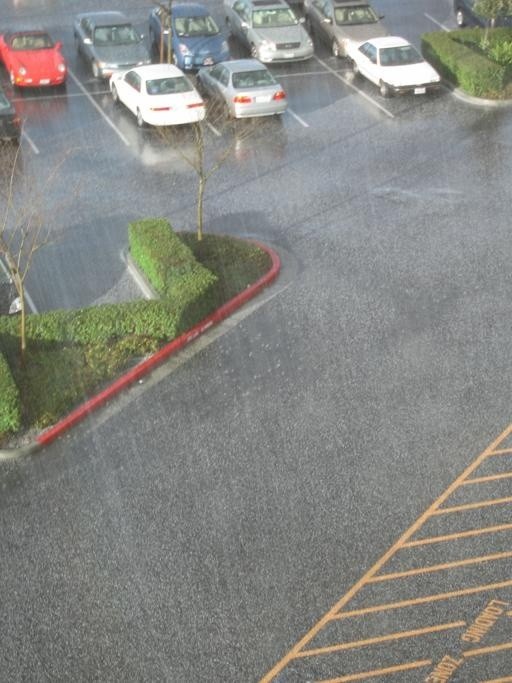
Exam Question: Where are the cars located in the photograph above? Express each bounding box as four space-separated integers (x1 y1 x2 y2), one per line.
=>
0 28 68 89
0 90 22 143
73 10 151 80
223 1 314 64
302 2 391 59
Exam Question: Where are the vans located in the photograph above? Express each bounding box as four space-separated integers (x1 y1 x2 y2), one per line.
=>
347 36 442 99
148 3 229 73
194 58 288 120
108 64 206 129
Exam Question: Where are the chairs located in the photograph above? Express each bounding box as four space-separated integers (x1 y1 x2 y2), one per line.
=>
95 27 128 41
175 18 205 32
252 7 367 25
12 38 44 49
